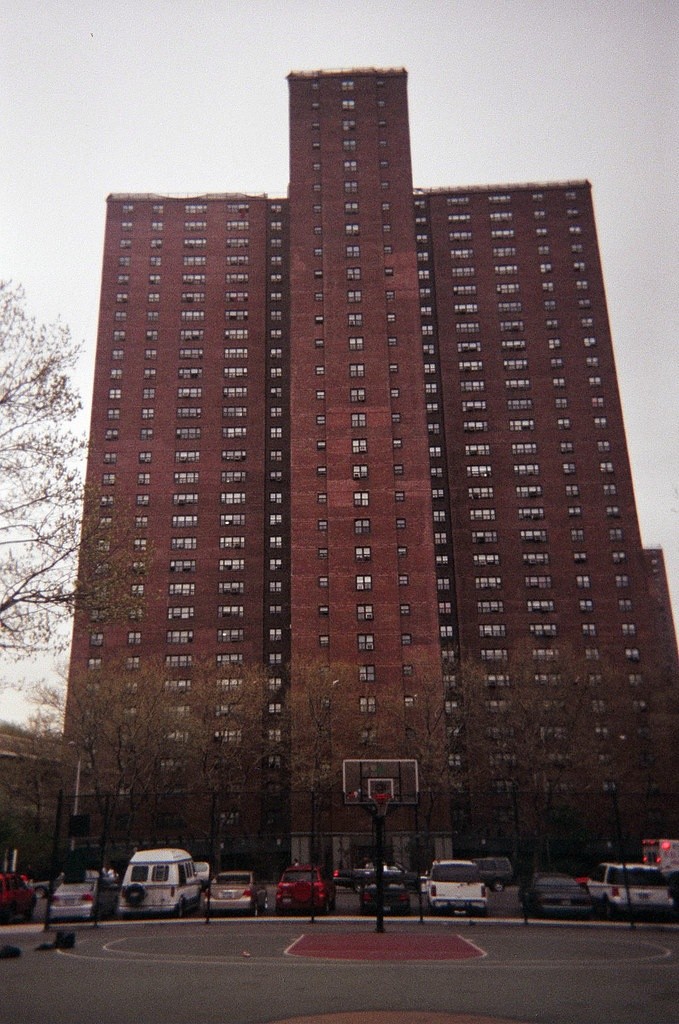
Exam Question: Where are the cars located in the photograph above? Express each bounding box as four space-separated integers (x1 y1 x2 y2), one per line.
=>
194 861 217 887
203 870 269 917
333 859 434 892
519 870 595 918
359 869 411 915
31 872 99 898
49 879 102 921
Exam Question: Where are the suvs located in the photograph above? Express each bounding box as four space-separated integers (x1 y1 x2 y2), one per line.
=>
424 858 488 914
0 873 38 921
275 865 348 913
587 863 678 921
477 857 516 893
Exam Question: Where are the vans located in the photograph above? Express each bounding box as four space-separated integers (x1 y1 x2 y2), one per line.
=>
118 849 203 920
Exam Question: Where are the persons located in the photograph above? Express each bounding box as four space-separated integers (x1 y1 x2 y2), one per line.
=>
293 859 300 866
